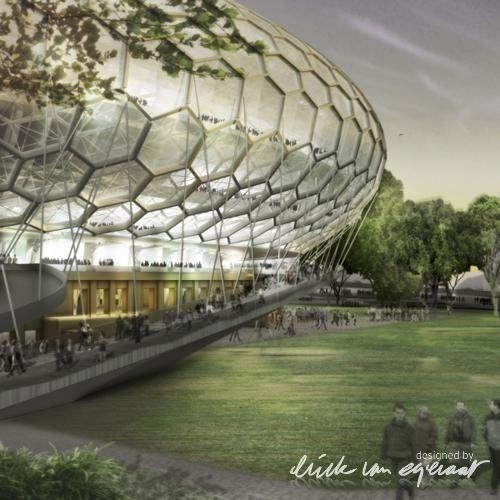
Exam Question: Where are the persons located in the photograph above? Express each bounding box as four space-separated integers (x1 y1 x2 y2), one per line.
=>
413 406 439 490
380 403 417 500
442 399 481 489
481 399 500 493
